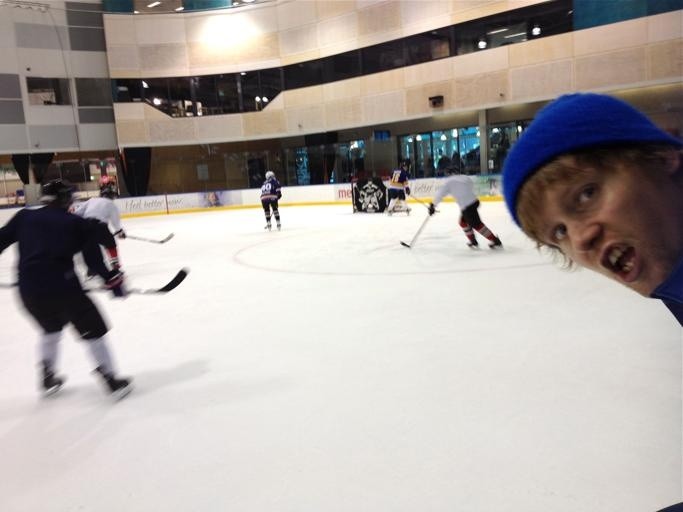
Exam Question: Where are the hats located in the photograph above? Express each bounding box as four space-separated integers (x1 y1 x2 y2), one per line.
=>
502 93 681 228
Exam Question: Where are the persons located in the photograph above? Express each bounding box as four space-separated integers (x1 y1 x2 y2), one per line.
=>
435 140 510 178
260 171 282 230
0 174 130 393
501 92 683 328
429 166 501 248
383 159 412 217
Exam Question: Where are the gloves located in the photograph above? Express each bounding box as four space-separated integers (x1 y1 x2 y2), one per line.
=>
429 203 435 215
118 230 126 238
406 187 410 195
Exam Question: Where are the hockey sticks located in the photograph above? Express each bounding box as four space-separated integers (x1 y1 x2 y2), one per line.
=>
126 232 175 244
399 212 430 248
83 269 187 293
411 196 441 214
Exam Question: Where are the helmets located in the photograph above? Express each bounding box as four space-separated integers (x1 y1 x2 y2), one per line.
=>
101 187 116 197
265 171 274 179
41 180 75 210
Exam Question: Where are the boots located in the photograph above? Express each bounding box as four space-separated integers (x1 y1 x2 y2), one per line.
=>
467 239 477 246
42 363 62 389
95 366 129 390
489 237 500 247
